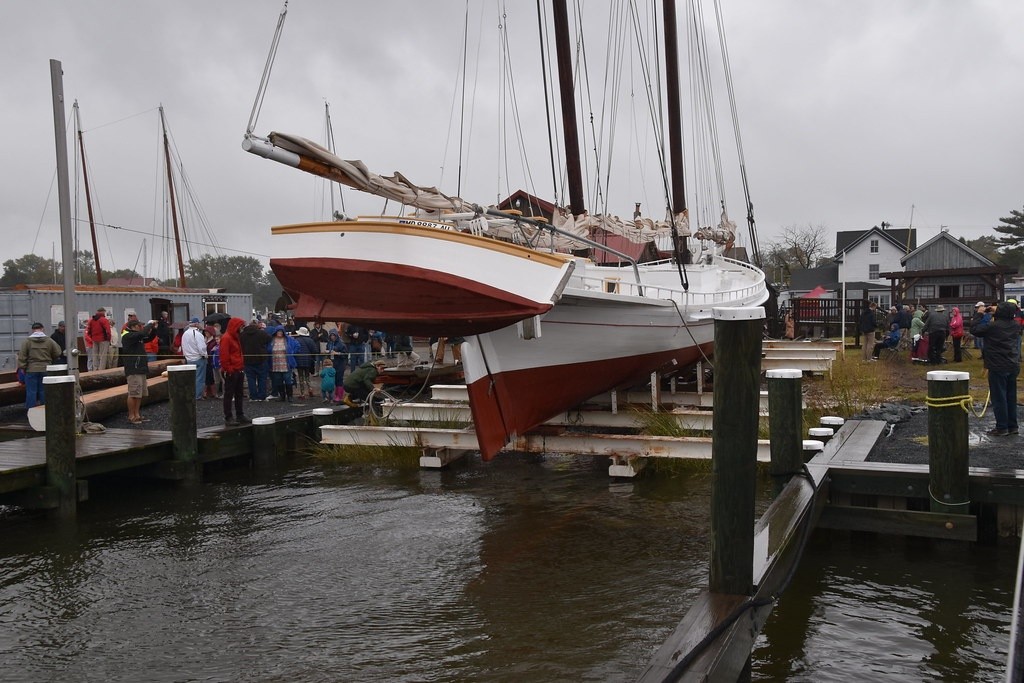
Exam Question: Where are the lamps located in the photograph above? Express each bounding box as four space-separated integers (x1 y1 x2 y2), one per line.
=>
516 199 522 207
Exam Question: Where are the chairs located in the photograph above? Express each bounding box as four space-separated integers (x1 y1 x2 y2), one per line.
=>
878 334 913 364
945 334 973 361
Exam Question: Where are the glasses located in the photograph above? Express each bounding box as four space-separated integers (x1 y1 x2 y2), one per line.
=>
128 314 134 317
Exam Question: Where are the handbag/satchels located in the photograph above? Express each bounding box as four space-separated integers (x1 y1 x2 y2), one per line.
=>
309 360 316 375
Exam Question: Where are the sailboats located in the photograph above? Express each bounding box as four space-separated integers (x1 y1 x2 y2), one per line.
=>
240 0 779 464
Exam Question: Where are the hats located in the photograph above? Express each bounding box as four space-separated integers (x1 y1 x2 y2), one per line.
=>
1007 299 1017 305
191 318 200 324
58 321 65 327
375 359 388 368
129 320 145 327
32 323 44 329
295 328 310 336
935 305 945 312
975 302 985 308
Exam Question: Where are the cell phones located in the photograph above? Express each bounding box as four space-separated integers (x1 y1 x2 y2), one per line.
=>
984 308 991 313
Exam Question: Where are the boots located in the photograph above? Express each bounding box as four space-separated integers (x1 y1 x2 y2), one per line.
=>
210 385 218 398
334 386 344 402
203 385 208 398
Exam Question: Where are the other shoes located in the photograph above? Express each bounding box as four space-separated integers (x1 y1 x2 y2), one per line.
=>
344 395 358 407
127 416 143 424
871 356 879 361
236 415 252 423
137 416 151 422
249 393 334 405
987 425 1018 437
224 419 239 426
434 359 443 365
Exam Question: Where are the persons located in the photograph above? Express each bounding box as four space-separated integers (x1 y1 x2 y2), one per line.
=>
970 302 1021 435
121 320 159 424
862 297 963 364
973 299 1024 377
219 317 251 425
50 321 67 376
19 322 61 409
85 306 466 406
